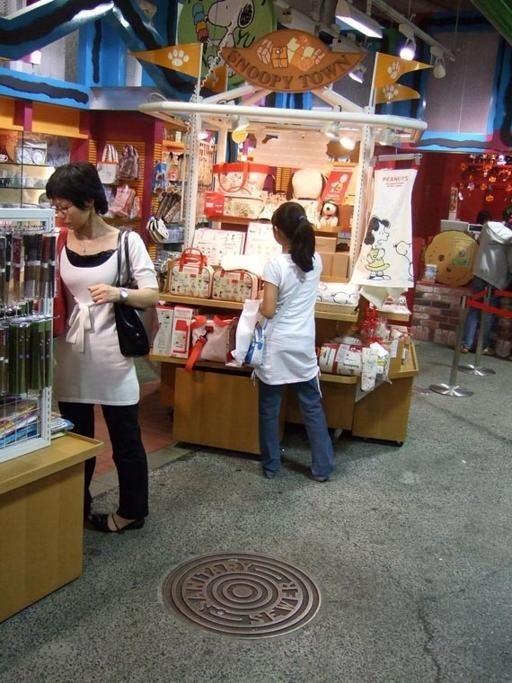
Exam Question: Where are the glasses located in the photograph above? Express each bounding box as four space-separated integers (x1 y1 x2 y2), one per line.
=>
49 205 73 213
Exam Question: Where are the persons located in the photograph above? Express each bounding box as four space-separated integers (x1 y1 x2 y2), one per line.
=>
242 202 336 483
460 205 512 356
45 161 160 534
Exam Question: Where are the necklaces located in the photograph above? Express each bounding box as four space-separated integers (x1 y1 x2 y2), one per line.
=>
69 222 108 255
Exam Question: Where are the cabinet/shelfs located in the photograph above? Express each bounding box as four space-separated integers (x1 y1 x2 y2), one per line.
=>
0 125 23 208
0 431 103 625
137 76 419 460
23 130 88 206
156 138 212 263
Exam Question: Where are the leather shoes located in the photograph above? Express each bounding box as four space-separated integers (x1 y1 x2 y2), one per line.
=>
88 514 145 532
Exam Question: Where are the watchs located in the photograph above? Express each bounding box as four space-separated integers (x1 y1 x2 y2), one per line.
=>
119 287 129 304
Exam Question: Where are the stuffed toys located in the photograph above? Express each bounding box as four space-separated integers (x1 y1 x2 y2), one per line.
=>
319 197 339 228
283 166 330 225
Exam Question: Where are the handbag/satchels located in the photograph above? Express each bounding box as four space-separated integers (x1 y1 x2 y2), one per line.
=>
169 248 257 302
113 283 160 357
185 299 266 372
96 144 139 220
214 162 270 219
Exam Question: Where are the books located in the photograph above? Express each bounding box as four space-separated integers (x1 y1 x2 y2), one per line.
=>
0 392 75 446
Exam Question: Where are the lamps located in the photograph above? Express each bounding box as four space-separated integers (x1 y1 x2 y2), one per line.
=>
333 0 457 85
